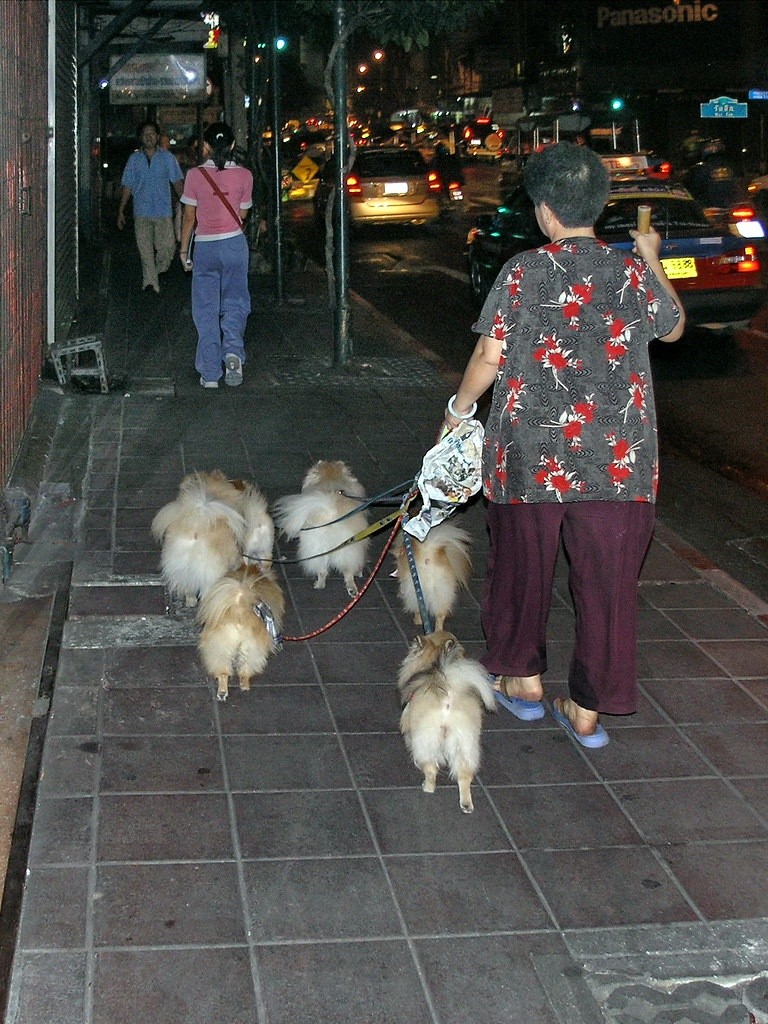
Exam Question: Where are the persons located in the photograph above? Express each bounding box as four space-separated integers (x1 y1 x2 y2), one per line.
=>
442 140 686 750
179 123 254 387
118 122 189 295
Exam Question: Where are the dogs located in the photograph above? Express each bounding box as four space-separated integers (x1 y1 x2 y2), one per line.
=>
397 629 496 815
272 457 369 597
388 504 471 633
150 468 285 703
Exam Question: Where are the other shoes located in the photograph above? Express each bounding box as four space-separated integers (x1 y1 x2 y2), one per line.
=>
225 353 243 387
200 377 218 388
143 283 160 294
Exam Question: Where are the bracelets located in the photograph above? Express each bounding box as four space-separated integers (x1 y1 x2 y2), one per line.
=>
448 393 478 419
179 251 188 254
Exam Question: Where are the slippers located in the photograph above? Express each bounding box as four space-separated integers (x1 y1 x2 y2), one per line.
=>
485 675 545 720
552 697 610 748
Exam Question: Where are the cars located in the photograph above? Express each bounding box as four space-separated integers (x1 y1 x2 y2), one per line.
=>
312 143 443 230
463 154 768 322
261 113 510 203
